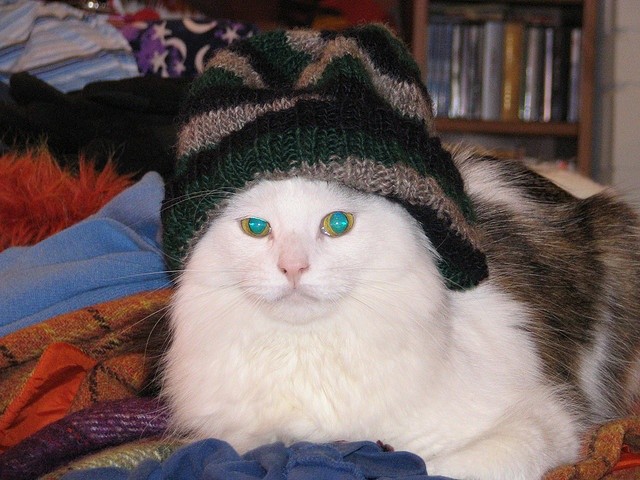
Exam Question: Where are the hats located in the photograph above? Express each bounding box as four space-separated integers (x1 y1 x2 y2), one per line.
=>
162 23 489 291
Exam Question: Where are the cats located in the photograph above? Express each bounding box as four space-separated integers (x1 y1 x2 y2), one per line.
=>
155 140 640 480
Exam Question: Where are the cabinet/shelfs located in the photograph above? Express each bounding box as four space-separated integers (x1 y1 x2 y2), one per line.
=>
412 0 597 178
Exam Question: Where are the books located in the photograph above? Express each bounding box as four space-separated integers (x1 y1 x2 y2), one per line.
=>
423 19 581 125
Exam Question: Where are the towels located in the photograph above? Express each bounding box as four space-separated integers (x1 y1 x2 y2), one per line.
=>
0 170 167 339
57 436 456 480
